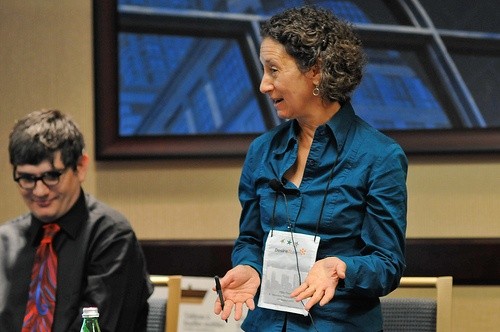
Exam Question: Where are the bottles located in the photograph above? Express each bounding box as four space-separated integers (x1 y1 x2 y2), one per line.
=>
80 307 101 332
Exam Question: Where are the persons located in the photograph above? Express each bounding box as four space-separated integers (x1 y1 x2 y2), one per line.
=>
0 109 154 332
212 5 408 332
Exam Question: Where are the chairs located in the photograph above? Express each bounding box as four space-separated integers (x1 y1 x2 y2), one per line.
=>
379 275 452 332
145 275 182 332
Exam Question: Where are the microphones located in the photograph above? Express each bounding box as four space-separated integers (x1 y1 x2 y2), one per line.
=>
268 178 283 193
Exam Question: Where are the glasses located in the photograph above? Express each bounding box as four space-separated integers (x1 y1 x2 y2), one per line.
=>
13 166 69 189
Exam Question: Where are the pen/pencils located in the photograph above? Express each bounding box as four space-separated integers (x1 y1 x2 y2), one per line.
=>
214 276 228 324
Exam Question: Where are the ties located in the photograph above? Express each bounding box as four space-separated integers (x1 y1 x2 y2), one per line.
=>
20 225 60 332
